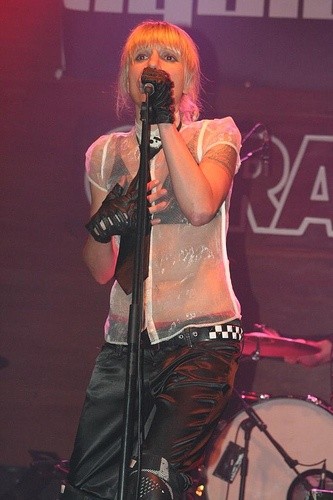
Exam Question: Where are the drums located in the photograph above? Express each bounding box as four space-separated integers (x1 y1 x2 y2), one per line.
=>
197 393 333 500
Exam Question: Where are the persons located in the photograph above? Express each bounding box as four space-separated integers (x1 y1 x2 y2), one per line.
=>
66 20 242 499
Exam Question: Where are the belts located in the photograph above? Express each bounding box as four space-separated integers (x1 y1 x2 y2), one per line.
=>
109 319 244 344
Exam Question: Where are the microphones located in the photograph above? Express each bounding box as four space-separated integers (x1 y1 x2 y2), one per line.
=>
143 83 155 96
